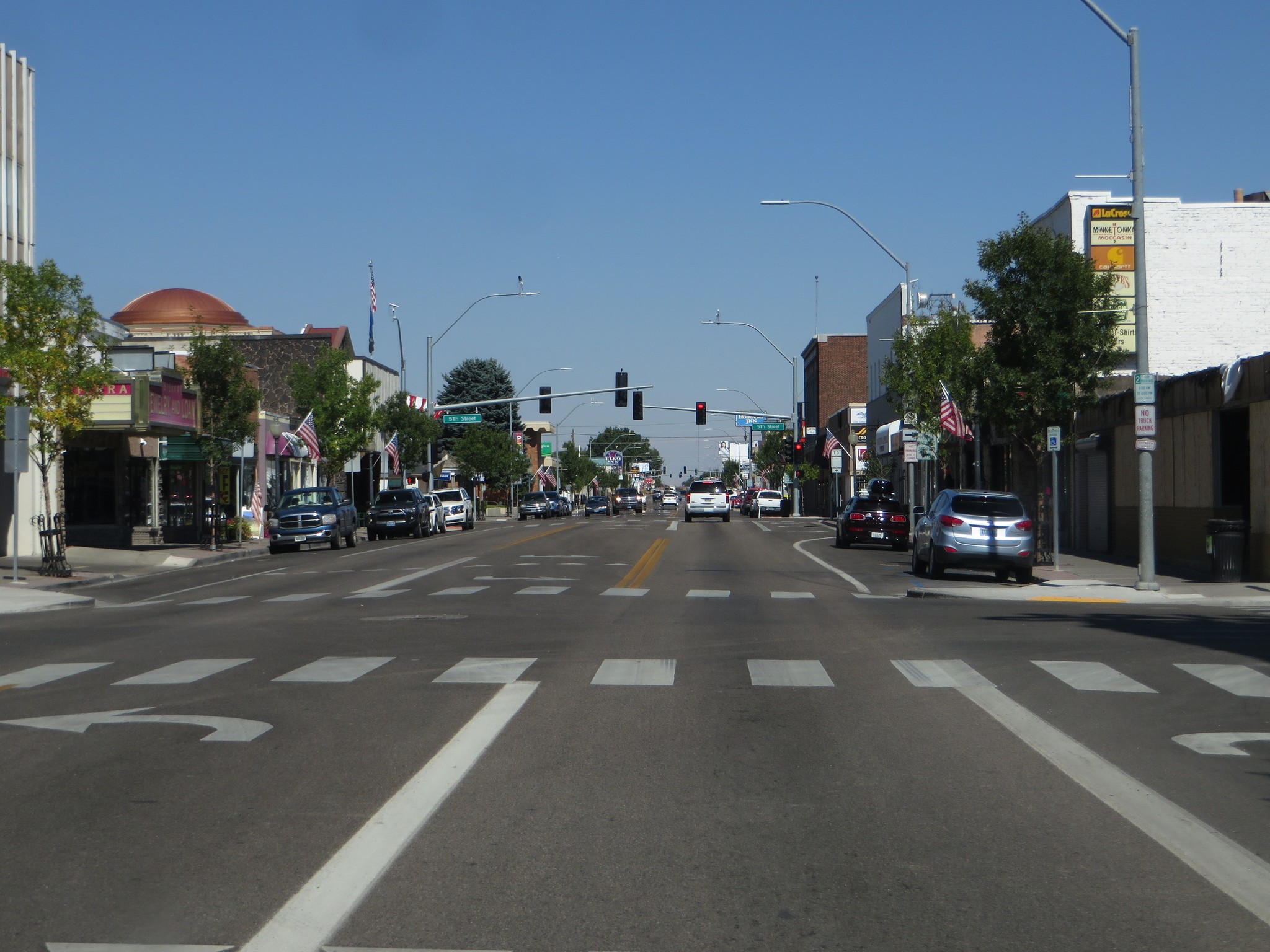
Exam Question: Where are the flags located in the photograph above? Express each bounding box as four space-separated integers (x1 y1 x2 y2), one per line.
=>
940 387 974 440
761 466 772 482
538 465 546 487
251 478 262 526
385 434 400 475
734 474 739 483
297 412 321 461
545 468 557 486
822 430 839 459
735 481 739 487
593 477 599 487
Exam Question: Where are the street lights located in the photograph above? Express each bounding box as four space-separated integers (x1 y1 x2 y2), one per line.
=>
701 321 799 515
761 199 915 549
269 416 283 501
703 388 768 487
427 291 540 489
510 367 572 514
366 436 376 504
742 475 746 497
555 401 604 491
750 471 755 487
848 430 858 496
527 465 533 492
732 477 736 488
589 424 664 497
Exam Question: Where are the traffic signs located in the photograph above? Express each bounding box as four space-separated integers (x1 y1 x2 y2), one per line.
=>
1046 427 1061 452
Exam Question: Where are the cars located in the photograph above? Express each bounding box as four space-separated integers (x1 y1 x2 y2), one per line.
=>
584 496 611 517
730 488 785 516
560 497 572 515
662 493 677 506
652 487 682 501
419 492 446 535
834 479 910 551
681 480 733 522
913 487 1036 585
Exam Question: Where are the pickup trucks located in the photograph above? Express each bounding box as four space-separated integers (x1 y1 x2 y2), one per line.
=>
263 487 358 551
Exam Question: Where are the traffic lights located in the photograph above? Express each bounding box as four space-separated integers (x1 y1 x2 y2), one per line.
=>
696 402 706 425
694 468 698 475
794 442 804 464
700 475 702 479
795 470 802 478
407 476 416 484
684 466 687 474
783 439 794 462
633 391 643 420
690 475 692 479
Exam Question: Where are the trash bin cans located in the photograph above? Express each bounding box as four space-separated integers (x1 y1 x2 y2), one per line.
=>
1203 519 1246 582
780 499 803 515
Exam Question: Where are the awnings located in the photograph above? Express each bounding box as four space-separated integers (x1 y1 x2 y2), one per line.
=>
266 432 308 457
876 420 901 456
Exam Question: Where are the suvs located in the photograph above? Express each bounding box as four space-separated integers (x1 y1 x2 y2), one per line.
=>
428 486 474 532
365 487 430 542
518 491 565 519
611 488 647 514
